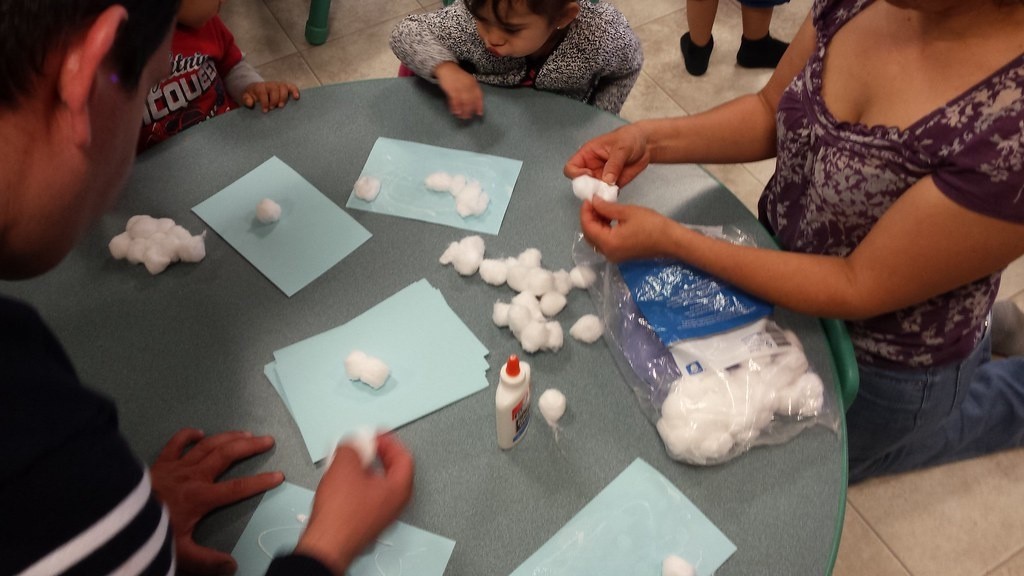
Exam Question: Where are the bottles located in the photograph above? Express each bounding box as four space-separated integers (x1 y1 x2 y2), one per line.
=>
495 354 532 450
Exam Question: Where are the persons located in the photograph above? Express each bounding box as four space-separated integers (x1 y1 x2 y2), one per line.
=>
387 0 645 123
680 0 791 77
134 0 300 152
564 0 1024 485
0 0 417 576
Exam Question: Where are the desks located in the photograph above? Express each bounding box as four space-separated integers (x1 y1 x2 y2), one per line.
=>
0 76 850 576
